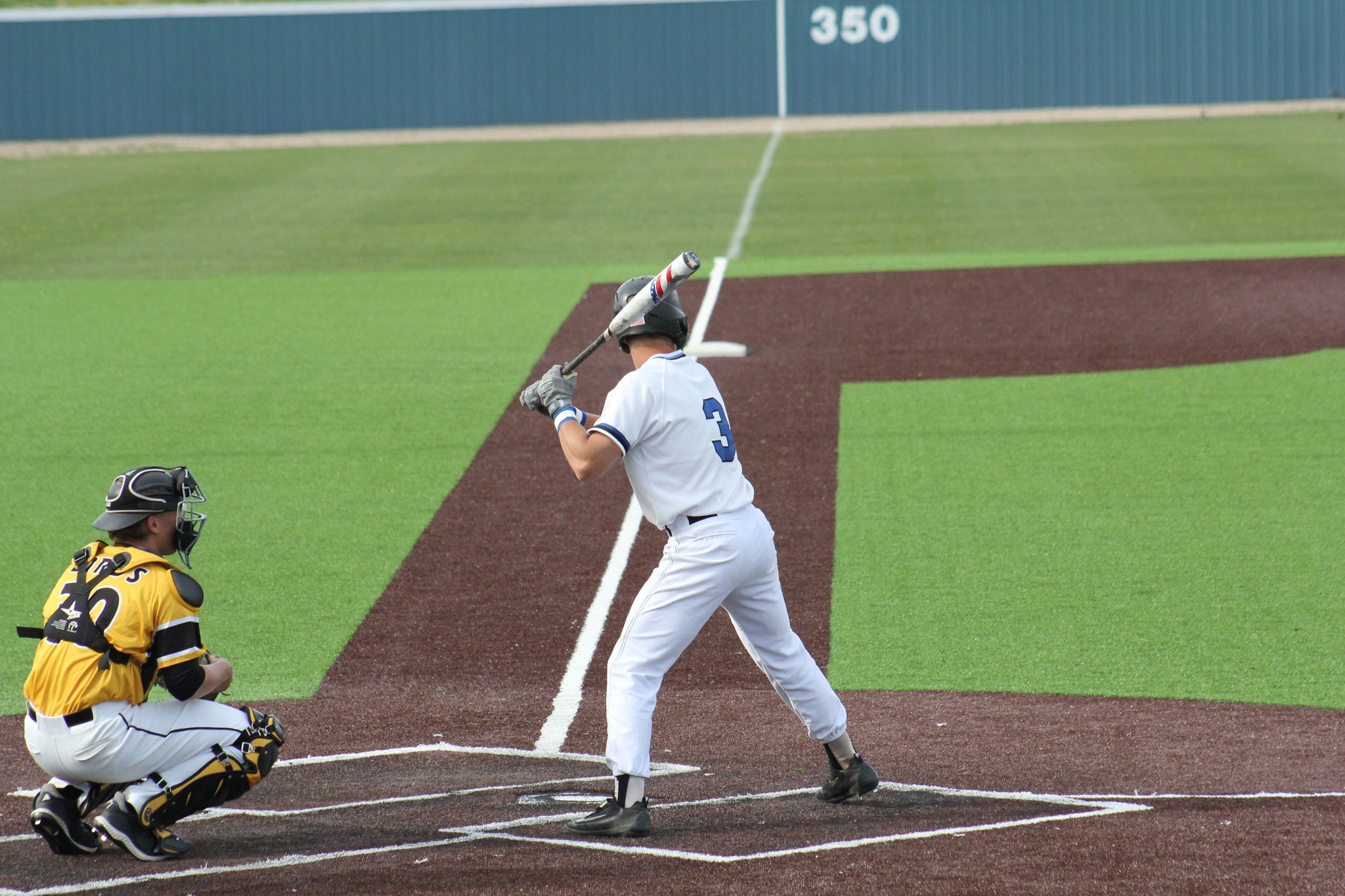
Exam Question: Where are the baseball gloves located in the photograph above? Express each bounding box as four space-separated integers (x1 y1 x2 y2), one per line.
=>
154 648 220 701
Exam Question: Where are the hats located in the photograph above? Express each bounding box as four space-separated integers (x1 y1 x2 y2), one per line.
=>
92 466 182 533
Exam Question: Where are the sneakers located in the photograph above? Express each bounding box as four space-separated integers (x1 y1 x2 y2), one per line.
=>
29 782 102 855
94 790 172 862
565 793 653 838
815 752 883 804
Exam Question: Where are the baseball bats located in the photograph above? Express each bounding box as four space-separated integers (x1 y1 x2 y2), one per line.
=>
524 251 700 409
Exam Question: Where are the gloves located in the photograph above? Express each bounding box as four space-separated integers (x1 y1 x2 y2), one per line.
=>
537 361 579 432
520 380 586 427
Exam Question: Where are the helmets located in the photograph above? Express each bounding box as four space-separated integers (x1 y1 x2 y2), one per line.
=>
612 275 689 351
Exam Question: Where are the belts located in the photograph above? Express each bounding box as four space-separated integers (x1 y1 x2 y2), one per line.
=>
664 513 718 537
25 702 93 727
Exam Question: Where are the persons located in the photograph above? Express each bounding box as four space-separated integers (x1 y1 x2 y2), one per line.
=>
519 274 879 838
24 466 285 863
